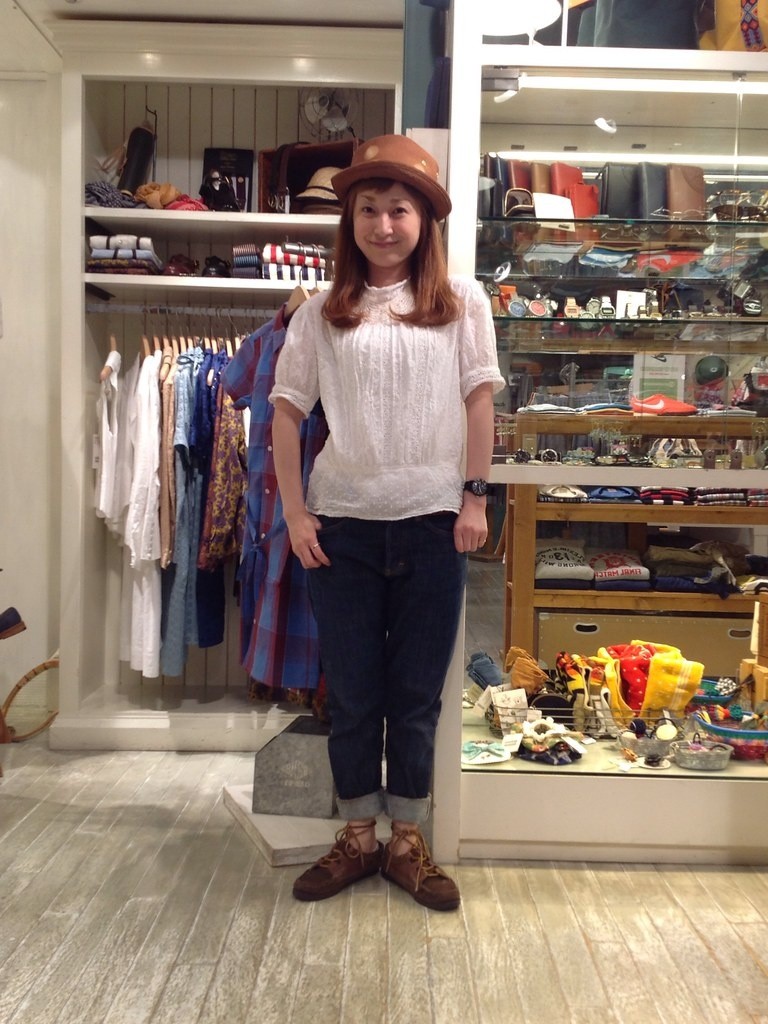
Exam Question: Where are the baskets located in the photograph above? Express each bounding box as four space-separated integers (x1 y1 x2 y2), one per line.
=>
667 733 733 769
618 729 679 758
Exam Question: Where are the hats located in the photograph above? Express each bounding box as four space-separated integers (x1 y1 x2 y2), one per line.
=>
332 133 453 223
296 166 341 202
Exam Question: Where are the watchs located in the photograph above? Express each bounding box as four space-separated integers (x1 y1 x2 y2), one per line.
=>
536 449 561 462
462 477 488 497
486 280 764 330
510 449 531 464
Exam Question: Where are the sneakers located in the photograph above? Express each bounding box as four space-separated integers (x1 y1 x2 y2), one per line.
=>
382 824 461 912
292 820 383 902
630 393 698 416
637 251 704 272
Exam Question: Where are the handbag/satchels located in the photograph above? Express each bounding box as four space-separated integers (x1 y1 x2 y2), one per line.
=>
484 154 708 221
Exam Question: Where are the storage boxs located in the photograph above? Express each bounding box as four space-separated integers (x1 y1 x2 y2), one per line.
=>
634 355 685 404
258 138 364 214
204 148 253 213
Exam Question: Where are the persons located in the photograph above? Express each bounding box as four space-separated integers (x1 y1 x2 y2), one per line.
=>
267 134 504 912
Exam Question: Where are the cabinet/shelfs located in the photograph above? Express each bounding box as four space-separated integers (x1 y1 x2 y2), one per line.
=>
429 0 767 867
43 20 403 750
505 417 768 680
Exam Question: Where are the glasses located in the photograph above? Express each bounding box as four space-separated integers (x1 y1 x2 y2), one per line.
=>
712 204 768 221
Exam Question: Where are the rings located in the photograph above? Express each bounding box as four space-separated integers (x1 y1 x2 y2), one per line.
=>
310 543 319 550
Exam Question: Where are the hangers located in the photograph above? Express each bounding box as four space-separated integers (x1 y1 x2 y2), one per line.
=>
284 243 321 317
140 301 268 386
100 304 116 381
